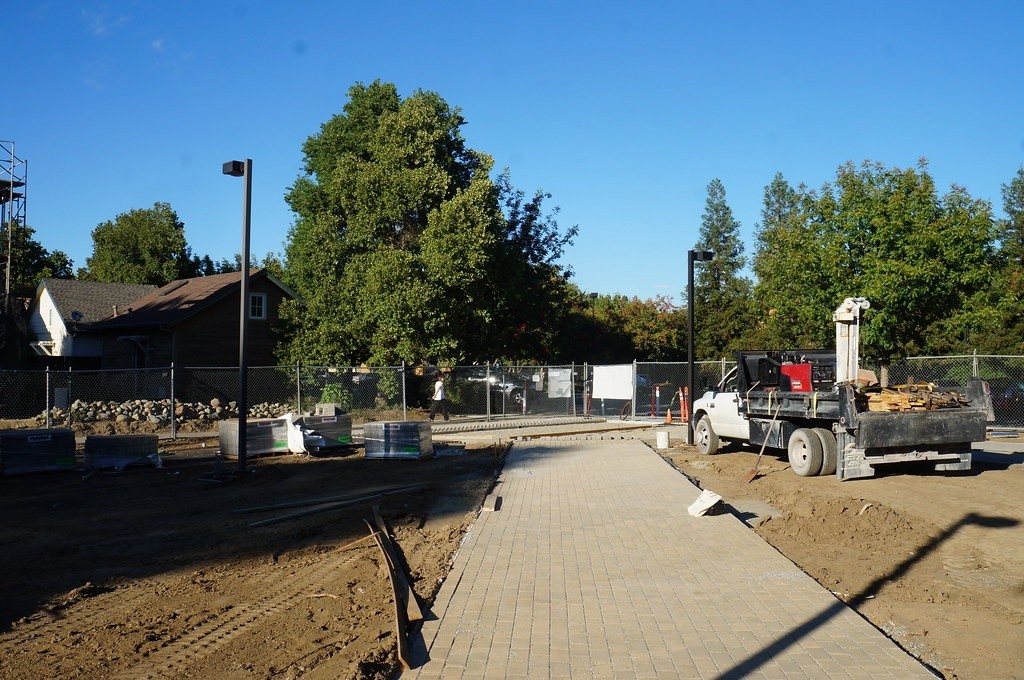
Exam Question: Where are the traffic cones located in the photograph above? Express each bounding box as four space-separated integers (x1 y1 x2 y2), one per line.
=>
666 409 672 423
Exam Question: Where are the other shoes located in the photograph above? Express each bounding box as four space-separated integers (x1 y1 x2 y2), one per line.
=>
443 420 451 422
427 418 434 422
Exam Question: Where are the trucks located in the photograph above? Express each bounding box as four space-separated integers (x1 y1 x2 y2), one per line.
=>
692 351 995 480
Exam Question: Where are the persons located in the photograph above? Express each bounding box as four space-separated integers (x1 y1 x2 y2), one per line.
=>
427 375 452 422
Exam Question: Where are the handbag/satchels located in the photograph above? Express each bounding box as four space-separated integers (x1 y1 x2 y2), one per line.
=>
687 489 723 518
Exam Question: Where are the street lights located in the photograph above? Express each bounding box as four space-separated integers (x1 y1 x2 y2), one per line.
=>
222 159 253 472
688 250 714 445
590 292 598 328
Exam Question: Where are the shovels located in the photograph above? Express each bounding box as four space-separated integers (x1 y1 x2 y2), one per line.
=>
744 406 781 483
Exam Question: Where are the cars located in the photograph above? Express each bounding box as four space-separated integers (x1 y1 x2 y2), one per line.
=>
989 377 1024 426
468 367 540 405
